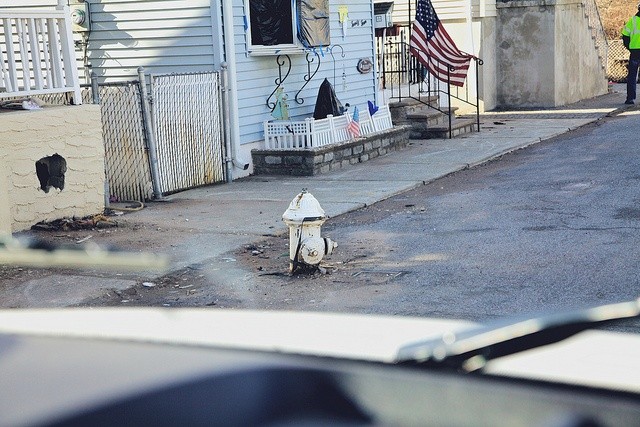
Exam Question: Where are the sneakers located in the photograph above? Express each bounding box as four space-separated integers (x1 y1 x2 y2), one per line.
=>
625 99 634 104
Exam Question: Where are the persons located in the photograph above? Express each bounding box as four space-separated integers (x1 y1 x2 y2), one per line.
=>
621 3 640 104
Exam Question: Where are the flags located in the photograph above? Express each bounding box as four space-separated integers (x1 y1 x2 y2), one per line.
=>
410 0 475 86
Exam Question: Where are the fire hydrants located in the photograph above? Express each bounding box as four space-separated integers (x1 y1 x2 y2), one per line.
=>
281 187 339 274
